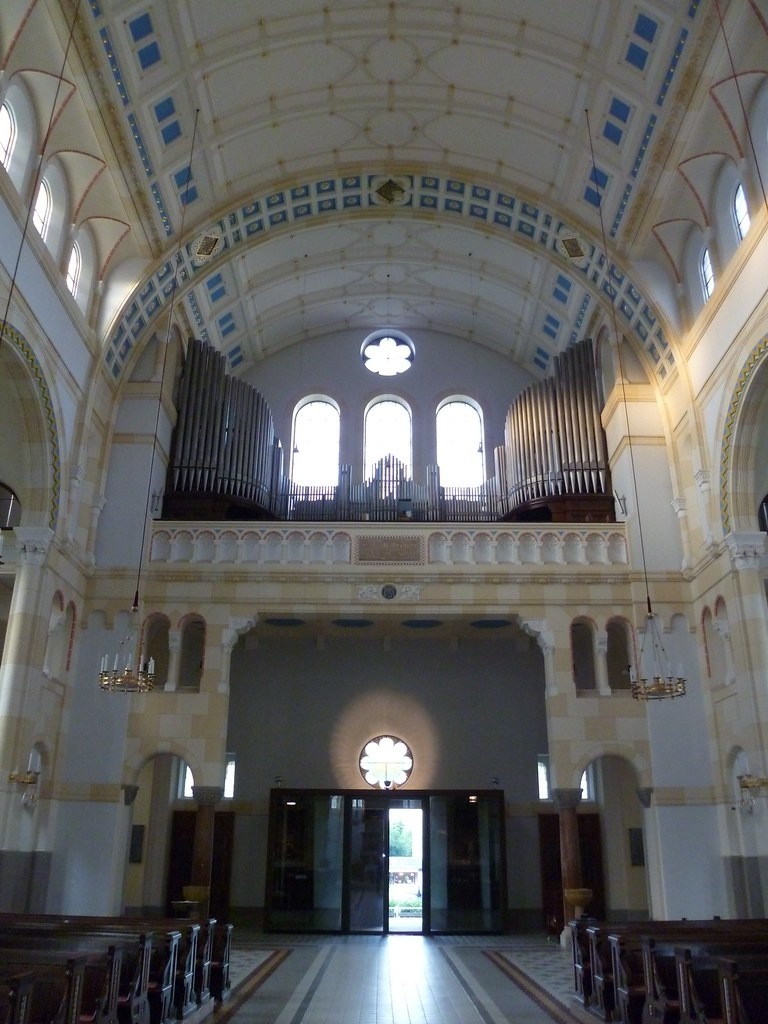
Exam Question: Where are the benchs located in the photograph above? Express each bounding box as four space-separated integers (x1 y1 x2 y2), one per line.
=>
0 914 233 1024
567 920 768 1024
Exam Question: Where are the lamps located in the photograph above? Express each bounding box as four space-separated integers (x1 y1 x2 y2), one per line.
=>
97 106 200 697
8 751 42 808
731 754 759 810
276 777 284 787
385 780 391 788
488 776 499 790
582 108 686 701
